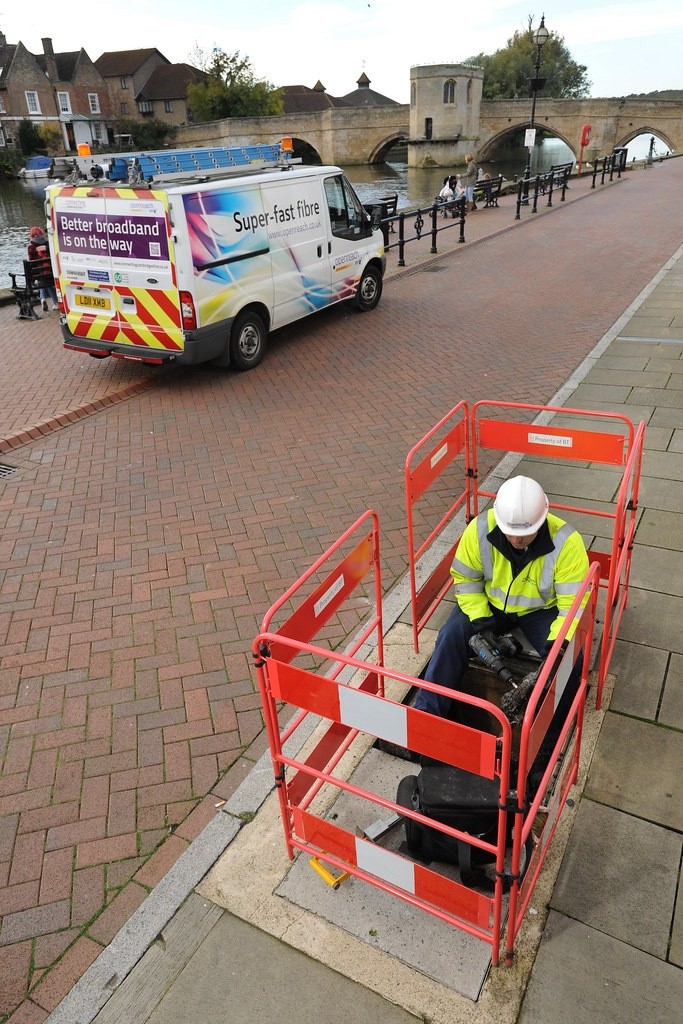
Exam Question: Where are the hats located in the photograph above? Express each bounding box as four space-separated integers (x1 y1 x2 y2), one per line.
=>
30 227 44 240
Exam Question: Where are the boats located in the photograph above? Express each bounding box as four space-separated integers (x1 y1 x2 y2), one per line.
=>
18 155 51 179
386 161 409 172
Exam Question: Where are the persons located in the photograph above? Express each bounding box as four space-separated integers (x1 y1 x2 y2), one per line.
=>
482 174 492 195
28 227 59 310
457 153 477 214
378 474 592 772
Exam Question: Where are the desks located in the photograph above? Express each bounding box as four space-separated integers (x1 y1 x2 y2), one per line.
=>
537 161 574 193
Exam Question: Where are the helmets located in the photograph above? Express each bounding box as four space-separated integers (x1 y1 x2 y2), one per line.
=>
493 475 549 537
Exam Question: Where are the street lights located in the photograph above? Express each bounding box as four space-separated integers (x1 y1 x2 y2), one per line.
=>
521 12 549 207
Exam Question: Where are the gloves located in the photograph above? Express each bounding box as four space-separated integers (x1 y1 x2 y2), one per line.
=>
535 647 569 691
480 629 511 658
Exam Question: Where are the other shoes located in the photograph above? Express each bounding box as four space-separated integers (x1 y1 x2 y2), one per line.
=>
41 298 48 311
52 304 60 310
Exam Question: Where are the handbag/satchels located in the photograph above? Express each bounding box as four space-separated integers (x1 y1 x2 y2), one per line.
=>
395 764 533 894
440 180 453 198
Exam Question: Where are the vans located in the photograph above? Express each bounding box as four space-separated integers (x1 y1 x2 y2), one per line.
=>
43 135 387 372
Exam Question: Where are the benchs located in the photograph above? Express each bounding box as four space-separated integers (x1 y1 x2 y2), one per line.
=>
449 173 504 212
378 191 399 233
8 257 55 320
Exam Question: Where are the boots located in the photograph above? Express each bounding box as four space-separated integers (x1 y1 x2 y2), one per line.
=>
464 201 473 216
